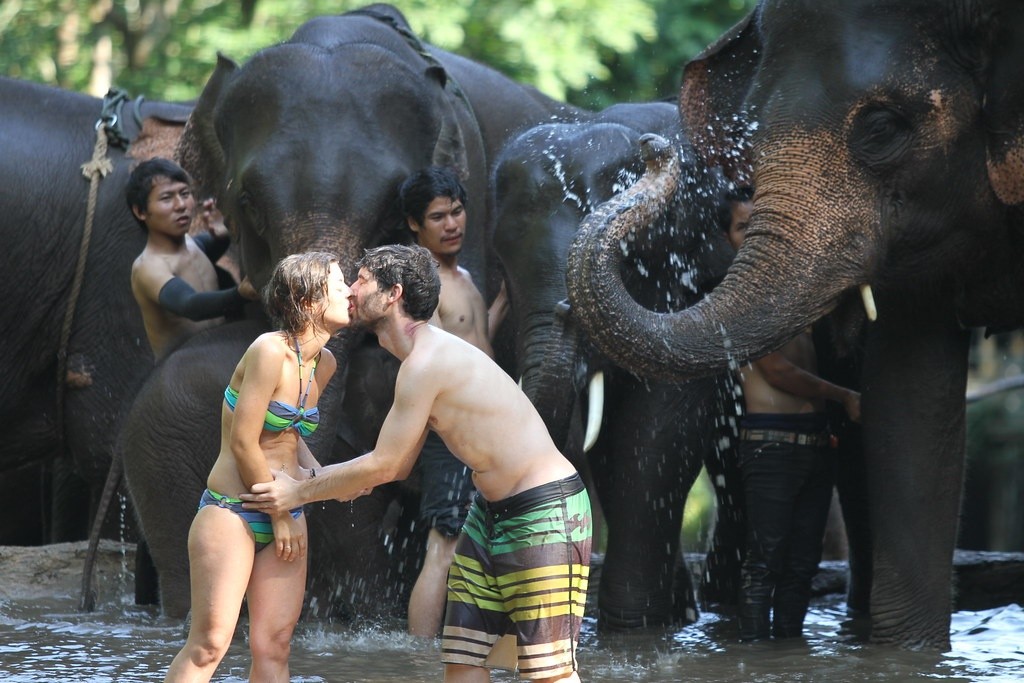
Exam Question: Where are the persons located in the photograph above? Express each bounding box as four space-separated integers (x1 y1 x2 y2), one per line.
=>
717 185 862 643
163 251 373 683
125 158 262 604
239 243 593 682
399 167 512 636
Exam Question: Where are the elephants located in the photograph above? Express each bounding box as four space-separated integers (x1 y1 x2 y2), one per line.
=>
1 0 1024 665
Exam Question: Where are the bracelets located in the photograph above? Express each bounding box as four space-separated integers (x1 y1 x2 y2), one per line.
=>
309 468 315 478
